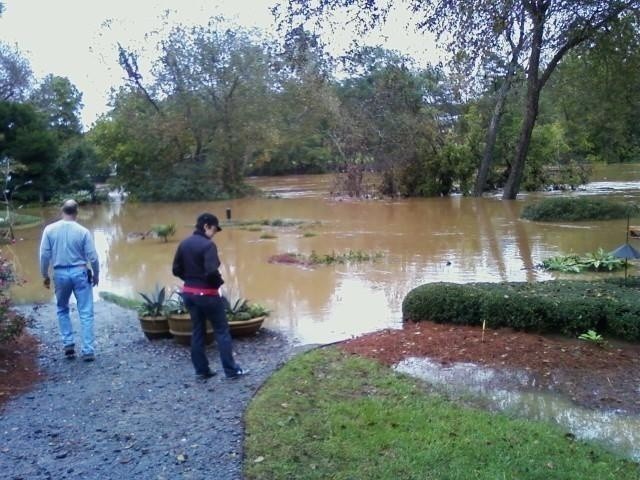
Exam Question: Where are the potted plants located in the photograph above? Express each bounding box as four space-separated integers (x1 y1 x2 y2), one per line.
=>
135 283 172 340
220 288 273 337
166 288 214 346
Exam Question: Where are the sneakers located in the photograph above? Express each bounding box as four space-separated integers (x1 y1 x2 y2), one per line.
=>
195 366 217 376
65 344 74 355
83 353 96 361
235 368 250 375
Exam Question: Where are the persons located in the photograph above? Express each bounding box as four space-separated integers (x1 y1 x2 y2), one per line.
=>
172 212 245 378
40 199 100 361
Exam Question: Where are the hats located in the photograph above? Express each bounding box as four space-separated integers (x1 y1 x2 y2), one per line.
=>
197 213 221 231
